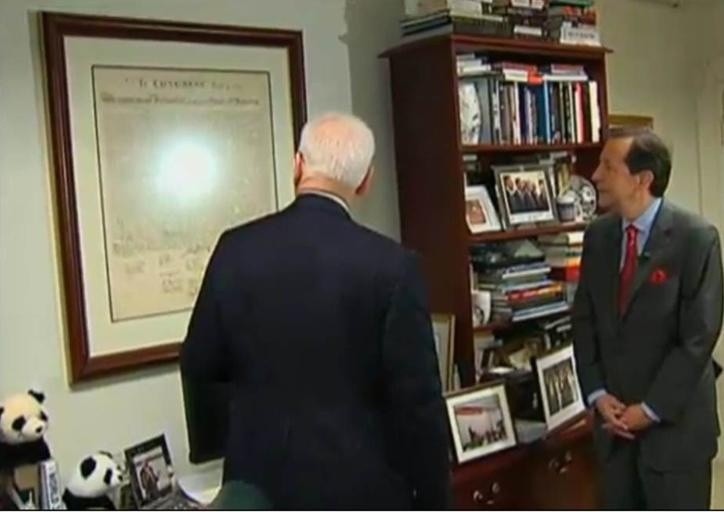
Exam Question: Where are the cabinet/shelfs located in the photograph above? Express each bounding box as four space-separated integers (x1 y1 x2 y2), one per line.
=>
372 31 621 427
449 413 615 509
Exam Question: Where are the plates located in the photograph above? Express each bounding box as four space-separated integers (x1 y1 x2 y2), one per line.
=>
565 173 598 220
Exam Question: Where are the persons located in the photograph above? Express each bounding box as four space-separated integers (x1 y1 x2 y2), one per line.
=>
497 172 550 215
140 459 161 501
572 126 724 511
179 112 454 510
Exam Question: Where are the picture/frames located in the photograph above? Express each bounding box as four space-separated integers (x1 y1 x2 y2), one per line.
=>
34 8 311 389
423 308 457 396
440 377 522 467
121 433 178 510
529 341 591 436
465 184 503 235
488 161 561 229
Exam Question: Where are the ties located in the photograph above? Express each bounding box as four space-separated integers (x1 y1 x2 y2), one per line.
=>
617 222 638 322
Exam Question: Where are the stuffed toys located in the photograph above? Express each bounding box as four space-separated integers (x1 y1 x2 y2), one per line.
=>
0 389 51 470
63 450 123 511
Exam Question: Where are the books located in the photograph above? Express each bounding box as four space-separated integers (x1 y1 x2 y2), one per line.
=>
470 227 591 326
401 0 610 145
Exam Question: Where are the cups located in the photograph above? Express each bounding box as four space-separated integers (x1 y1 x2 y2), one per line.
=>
557 198 585 225
471 289 491 328
